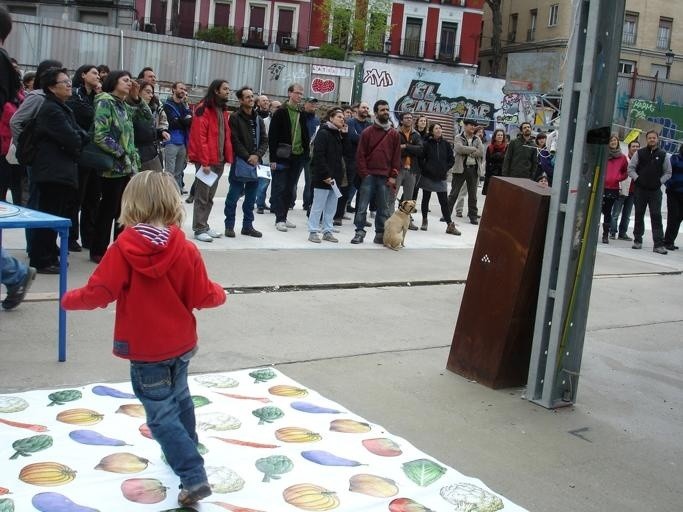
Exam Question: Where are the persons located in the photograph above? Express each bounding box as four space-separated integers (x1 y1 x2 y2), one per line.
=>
665 144 683 250
610 140 640 241
628 130 672 254
1 7 37 309
601 137 628 243
59 169 227 505
1 58 481 243
481 123 558 194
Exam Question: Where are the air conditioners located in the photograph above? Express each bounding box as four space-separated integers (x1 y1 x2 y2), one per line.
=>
281 36 291 46
144 23 157 33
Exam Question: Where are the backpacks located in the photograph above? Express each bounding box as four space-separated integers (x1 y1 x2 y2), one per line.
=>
15 103 63 168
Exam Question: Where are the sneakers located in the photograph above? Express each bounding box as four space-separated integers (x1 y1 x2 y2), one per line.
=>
193 233 212 242
1 266 36 309
206 228 222 238
240 226 261 237
27 240 102 274
224 227 235 237
257 188 418 244
186 195 194 203
177 482 211 505
608 231 679 254
420 213 480 235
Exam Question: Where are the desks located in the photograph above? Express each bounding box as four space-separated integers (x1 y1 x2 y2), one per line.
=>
1 203 73 362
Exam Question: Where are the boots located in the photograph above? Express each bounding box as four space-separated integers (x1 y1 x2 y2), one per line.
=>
602 223 609 243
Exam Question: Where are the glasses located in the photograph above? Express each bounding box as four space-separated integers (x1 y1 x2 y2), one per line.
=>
56 80 72 83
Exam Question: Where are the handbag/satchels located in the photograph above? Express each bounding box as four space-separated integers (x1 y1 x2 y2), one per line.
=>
276 143 291 159
78 123 120 171
353 175 360 189
231 156 257 183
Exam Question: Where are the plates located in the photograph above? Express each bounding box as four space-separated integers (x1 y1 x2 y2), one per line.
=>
0 205 20 218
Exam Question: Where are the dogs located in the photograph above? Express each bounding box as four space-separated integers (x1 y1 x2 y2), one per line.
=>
382 199 417 251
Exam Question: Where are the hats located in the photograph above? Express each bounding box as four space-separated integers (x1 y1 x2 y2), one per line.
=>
303 97 318 102
462 119 478 126
